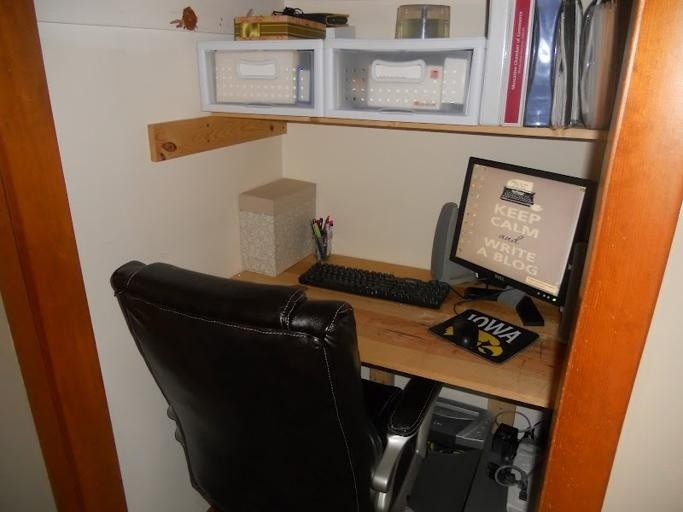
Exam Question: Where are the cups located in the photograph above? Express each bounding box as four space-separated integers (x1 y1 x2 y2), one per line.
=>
313 237 331 262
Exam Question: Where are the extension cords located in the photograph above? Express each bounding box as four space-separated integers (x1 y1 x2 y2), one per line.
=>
505 438 537 512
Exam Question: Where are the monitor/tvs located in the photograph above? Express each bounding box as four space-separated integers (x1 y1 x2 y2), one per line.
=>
449 156 592 328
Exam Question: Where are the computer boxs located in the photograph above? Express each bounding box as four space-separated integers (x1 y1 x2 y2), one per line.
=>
409 395 494 511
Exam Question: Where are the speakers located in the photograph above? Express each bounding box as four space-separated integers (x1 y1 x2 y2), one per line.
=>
428 202 474 285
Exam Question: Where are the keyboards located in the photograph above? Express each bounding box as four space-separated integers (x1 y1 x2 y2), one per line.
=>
299 261 450 310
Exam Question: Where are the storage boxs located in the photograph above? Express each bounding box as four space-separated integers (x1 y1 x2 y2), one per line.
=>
323 37 487 126
198 41 325 117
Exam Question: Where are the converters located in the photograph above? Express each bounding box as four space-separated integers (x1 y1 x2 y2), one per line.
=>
491 423 518 458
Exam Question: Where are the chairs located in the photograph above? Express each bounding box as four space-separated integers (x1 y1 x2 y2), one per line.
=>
110 260 445 511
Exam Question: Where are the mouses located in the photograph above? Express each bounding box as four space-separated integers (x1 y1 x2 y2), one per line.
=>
454 319 481 350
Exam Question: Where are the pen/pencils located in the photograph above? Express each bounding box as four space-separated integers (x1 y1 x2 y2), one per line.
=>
310 216 333 262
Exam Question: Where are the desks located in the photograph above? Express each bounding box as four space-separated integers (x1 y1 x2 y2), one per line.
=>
229 253 572 412
